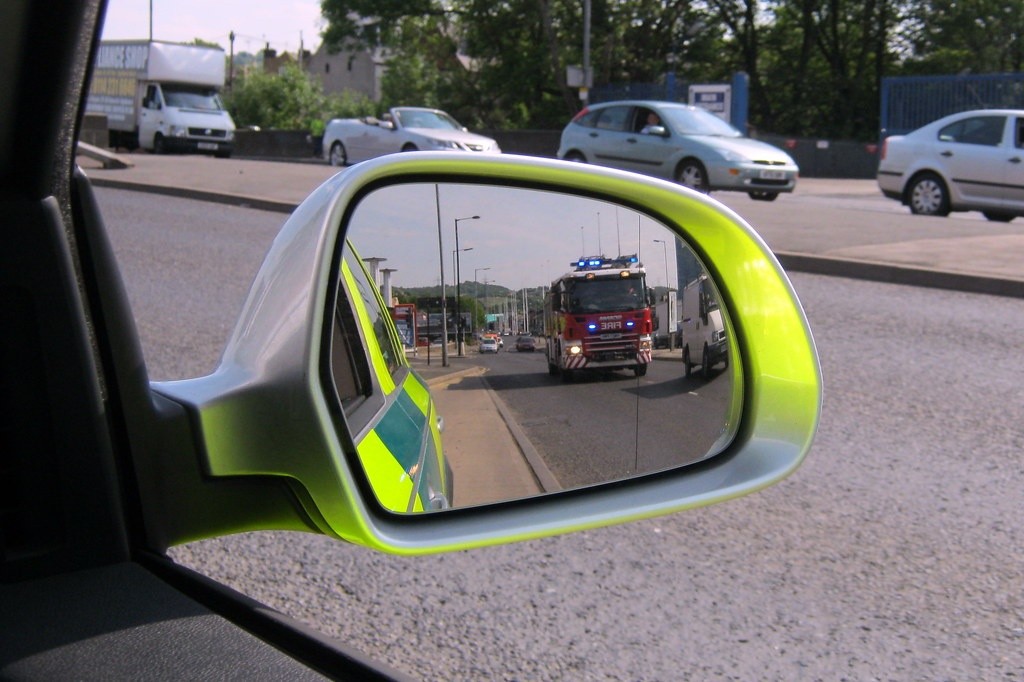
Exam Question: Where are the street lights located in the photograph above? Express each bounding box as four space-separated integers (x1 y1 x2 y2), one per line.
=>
452 247 474 351
472 267 491 343
454 215 480 357
653 239 670 290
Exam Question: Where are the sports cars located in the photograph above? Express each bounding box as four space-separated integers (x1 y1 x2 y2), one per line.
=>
321 106 503 167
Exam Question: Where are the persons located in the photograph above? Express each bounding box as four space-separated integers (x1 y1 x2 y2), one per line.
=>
641 114 660 134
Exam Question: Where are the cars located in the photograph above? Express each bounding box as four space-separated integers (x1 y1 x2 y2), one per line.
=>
480 333 504 353
556 99 799 202
877 108 1024 223
516 337 536 352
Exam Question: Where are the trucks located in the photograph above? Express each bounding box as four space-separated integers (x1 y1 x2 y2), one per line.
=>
85 39 236 158
681 274 731 379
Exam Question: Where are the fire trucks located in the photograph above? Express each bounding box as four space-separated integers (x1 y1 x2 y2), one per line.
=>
542 254 653 383
